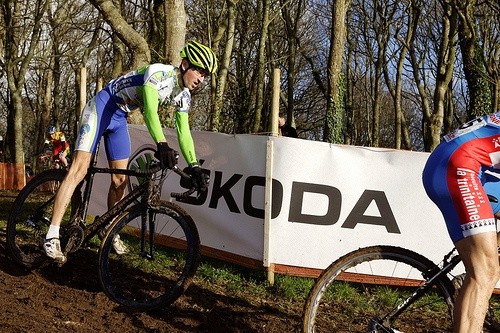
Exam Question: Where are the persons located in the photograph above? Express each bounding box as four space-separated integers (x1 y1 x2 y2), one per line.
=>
35 125 71 169
41 40 219 264
278 113 299 139
421 110 500 333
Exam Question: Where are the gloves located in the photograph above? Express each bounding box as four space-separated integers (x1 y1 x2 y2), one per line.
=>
190 167 210 192
157 142 179 169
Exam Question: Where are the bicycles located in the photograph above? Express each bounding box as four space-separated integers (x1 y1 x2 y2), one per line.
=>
38 149 70 194
5 129 209 312
300 193 500 333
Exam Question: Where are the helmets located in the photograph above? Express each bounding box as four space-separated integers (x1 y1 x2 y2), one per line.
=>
47 126 56 133
179 40 218 76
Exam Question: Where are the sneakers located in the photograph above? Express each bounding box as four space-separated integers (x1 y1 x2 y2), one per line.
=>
43 233 67 261
98 228 126 254
